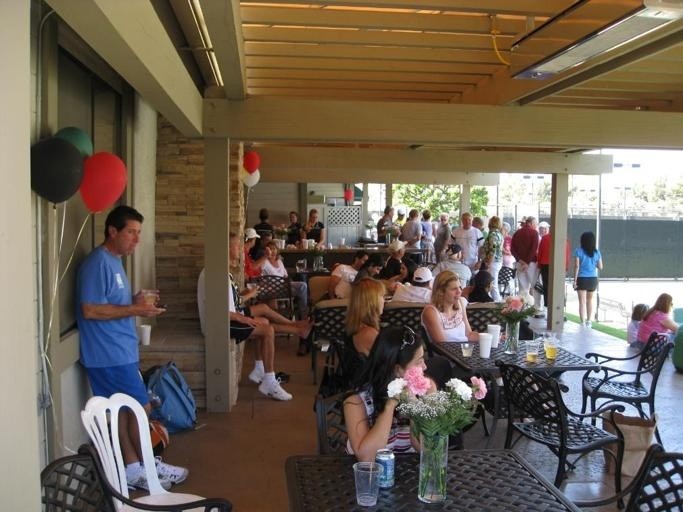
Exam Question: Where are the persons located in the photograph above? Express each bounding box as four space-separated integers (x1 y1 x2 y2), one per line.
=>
378 208 552 343
240 208 325 323
573 232 603 329
198 232 293 404
344 277 451 386
328 251 383 300
341 323 438 467
76 206 189 494
628 293 683 375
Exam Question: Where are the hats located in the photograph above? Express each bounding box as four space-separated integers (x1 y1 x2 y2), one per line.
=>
518 216 527 223
473 272 494 286
413 267 433 282
539 221 551 229
398 208 405 215
244 228 260 242
445 244 461 254
389 240 408 253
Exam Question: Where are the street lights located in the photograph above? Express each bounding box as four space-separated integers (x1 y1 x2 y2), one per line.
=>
523 176 545 207
580 189 596 206
613 162 640 213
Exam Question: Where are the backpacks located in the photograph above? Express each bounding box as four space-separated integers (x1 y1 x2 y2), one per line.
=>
144 361 197 433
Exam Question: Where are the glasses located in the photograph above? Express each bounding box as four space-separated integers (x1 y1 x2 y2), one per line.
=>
671 303 673 307
399 325 415 351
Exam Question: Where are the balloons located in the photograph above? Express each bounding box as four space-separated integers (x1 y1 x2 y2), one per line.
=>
54 126 94 158
243 150 260 173
80 151 129 212
244 169 261 188
344 188 354 201
32 137 83 209
242 167 248 176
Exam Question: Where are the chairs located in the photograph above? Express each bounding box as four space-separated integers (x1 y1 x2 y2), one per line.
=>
80 393 218 512
39 443 233 512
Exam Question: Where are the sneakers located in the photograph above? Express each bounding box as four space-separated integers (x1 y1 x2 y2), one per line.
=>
581 323 591 329
297 344 309 356
248 369 293 401
124 456 188 492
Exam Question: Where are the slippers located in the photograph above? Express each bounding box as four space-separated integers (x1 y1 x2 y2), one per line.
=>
300 319 314 340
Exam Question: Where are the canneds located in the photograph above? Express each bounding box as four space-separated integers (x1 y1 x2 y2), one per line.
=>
375 448 395 488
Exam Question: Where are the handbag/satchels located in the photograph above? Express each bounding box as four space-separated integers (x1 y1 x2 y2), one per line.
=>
313 373 341 414
534 282 544 294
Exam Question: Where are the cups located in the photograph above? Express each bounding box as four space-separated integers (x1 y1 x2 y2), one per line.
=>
140 325 152 346
461 344 475 358
142 289 156 304
274 238 315 250
543 331 557 360
338 237 345 246
488 324 501 348
247 282 256 290
525 341 539 363
478 332 492 359
312 255 323 272
353 461 385 507
296 257 308 273
